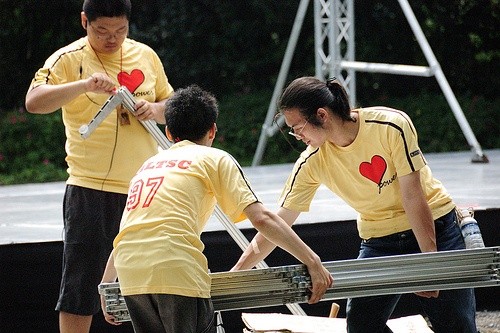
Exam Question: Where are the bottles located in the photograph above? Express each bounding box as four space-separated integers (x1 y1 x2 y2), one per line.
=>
460 209 485 249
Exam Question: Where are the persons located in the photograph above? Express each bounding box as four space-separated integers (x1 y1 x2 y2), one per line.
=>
100 83 334 333
229 77 476 333
25 0 176 333
